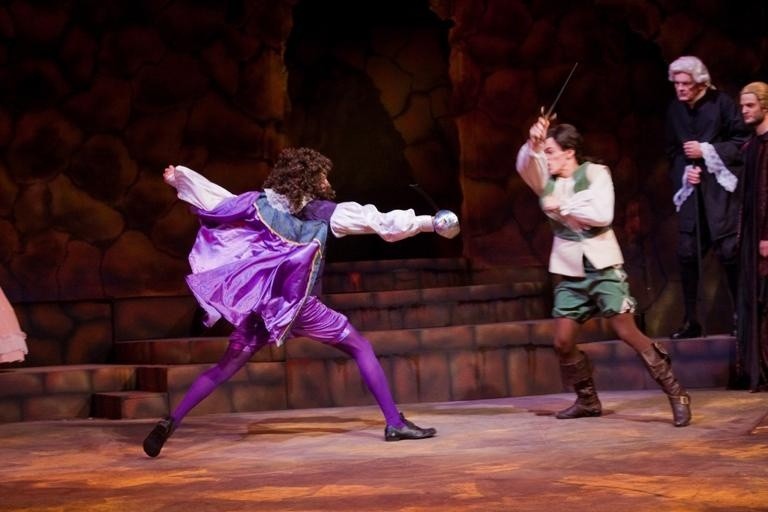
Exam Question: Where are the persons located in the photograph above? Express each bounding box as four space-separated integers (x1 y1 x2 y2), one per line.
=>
516 105 693 429
653 53 753 340
142 146 462 458
727 81 768 393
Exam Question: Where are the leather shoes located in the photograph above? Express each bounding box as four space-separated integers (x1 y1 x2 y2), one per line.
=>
671 321 702 338
730 323 737 336
143 416 173 457
385 412 435 441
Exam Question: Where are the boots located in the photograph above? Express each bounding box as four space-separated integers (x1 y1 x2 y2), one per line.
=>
555 352 601 418
649 357 691 426
